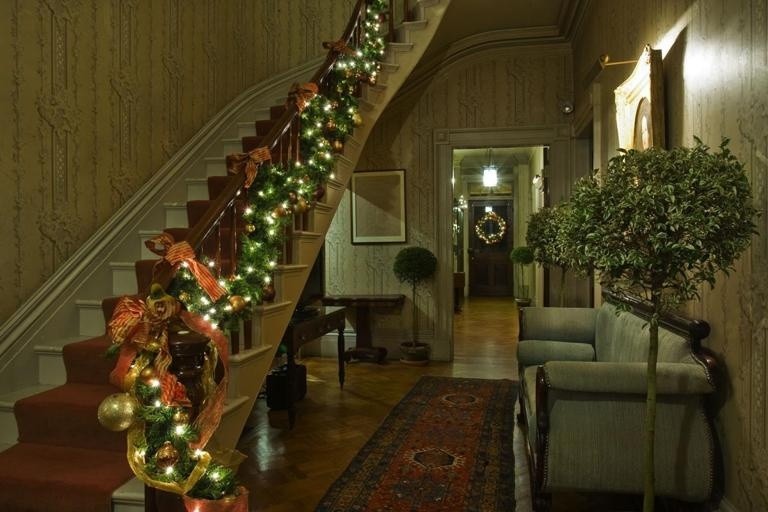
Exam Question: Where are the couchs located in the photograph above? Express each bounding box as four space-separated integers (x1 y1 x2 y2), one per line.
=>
516 289 732 512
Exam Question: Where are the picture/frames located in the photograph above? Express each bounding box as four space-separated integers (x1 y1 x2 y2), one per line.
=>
350 167 408 246
612 44 666 153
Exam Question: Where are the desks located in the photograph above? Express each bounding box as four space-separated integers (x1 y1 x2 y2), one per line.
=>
281 292 407 432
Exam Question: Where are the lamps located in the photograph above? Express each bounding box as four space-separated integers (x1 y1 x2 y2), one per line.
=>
483 148 496 189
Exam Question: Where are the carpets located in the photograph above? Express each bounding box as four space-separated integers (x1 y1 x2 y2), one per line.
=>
319 375 525 512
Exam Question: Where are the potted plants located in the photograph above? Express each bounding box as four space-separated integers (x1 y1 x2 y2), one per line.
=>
394 245 440 366
